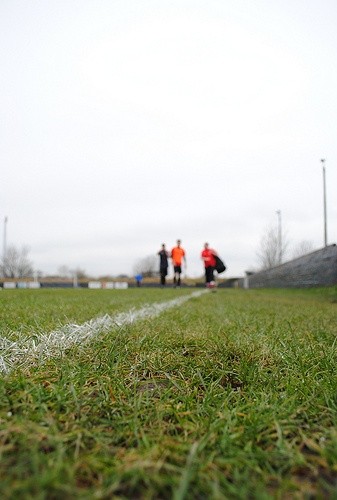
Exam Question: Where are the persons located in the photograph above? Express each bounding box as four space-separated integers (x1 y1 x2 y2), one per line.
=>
158 244 169 286
169 239 186 287
135 273 142 287
201 242 217 288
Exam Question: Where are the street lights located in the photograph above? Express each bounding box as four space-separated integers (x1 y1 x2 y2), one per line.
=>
276 209 280 265
319 158 327 247
